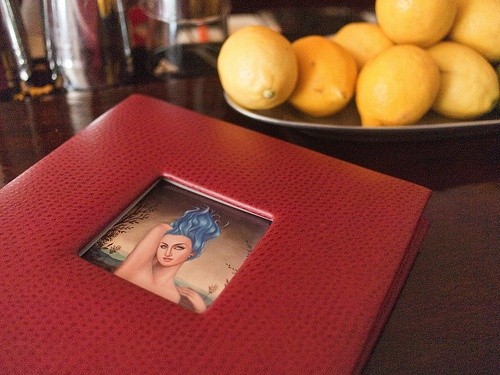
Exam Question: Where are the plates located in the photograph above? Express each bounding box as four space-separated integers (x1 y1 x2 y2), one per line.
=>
221 89 500 138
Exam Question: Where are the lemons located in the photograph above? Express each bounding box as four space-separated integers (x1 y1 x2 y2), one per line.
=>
217 0 499 127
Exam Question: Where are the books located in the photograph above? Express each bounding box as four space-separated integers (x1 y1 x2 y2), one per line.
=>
0 93 432 375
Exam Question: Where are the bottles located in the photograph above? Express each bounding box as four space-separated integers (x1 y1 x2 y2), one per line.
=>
41 0 133 92
0 0 34 99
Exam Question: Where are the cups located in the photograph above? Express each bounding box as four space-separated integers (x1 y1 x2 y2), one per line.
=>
128 1 230 85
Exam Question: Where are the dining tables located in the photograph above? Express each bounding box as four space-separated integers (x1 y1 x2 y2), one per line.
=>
1 0 499 375
